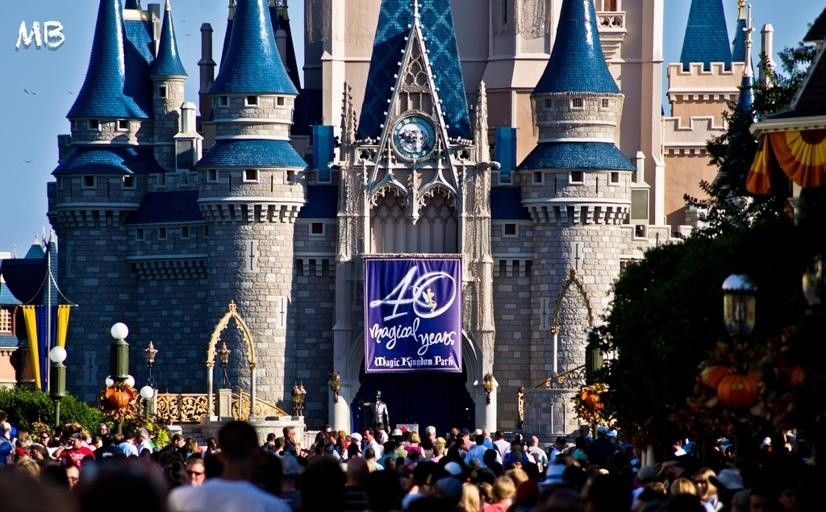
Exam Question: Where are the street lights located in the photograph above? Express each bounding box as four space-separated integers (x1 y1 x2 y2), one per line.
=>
584 325 606 441
139 385 153 420
297 379 307 449
105 322 135 446
802 241 826 512
49 346 67 427
289 380 302 449
721 261 759 471
143 341 158 423
216 342 232 423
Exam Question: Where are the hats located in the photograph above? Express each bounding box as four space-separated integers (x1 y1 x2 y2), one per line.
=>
708 468 745 492
392 428 403 436
69 433 82 441
435 437 446 446
637 465 657 480
597 426 618 438
437 477 463 500
579 425 590 432
351 432 363 440
763 443 772 448
621 444 633 453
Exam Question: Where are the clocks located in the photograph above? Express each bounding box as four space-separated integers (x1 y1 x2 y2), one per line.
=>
391 110 438 164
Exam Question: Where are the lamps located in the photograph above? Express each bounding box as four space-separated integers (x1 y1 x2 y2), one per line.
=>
483 368 498 404
329 367 342 406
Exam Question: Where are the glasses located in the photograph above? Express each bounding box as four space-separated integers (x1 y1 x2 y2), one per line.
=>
187 470 204 476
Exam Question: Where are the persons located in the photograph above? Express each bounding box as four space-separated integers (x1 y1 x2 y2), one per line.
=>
358 391 391 434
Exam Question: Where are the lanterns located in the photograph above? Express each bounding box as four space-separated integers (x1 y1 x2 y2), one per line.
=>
109 392 129 408
701 361 807 409
